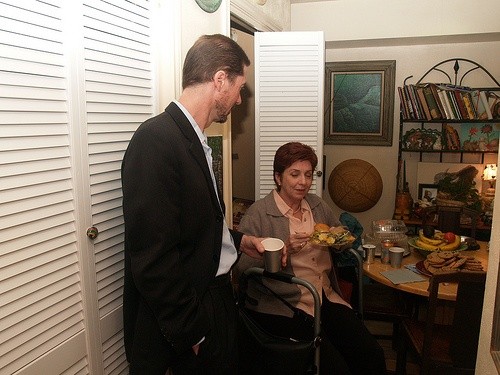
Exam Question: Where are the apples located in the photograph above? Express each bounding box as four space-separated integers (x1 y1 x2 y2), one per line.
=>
444 232 455 243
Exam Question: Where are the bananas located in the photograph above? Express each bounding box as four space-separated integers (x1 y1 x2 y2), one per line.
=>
417 229 460 251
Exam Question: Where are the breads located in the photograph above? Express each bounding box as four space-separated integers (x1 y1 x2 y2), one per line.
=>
313 223 330 233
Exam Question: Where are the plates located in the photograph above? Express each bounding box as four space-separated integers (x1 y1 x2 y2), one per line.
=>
408 237 468 258
415 260 433 277
307 232 358 247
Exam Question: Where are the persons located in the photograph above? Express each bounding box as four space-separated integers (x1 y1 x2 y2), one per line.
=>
120 33 288 375
231 142 386 375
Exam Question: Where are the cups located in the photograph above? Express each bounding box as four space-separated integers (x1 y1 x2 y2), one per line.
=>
389 247 405 268
363 244 376 264
260 238 284 273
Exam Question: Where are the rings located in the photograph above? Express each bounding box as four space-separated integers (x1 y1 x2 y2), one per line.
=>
301 243 303 247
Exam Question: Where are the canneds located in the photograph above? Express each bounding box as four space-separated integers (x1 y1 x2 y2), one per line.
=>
381 240 394 264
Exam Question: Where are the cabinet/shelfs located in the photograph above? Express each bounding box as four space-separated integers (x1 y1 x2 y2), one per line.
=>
392 58 500 238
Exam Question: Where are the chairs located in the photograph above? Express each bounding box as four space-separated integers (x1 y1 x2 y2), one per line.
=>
395 273 487 375
233 248 363 375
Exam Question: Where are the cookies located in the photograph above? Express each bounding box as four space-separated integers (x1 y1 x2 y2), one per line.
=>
423 250 483 274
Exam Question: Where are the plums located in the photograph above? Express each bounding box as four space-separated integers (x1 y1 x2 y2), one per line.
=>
423 226 434 237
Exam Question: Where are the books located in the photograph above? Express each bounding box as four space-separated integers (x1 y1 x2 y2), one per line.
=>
397 84 500 122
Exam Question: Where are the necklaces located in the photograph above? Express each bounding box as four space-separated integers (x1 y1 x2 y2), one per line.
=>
292 205 301 214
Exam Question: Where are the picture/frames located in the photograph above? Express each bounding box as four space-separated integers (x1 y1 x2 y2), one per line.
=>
324 60 396 147
418 183 439 202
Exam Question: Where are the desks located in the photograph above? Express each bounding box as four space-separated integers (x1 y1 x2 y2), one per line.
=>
363 239 489 322
